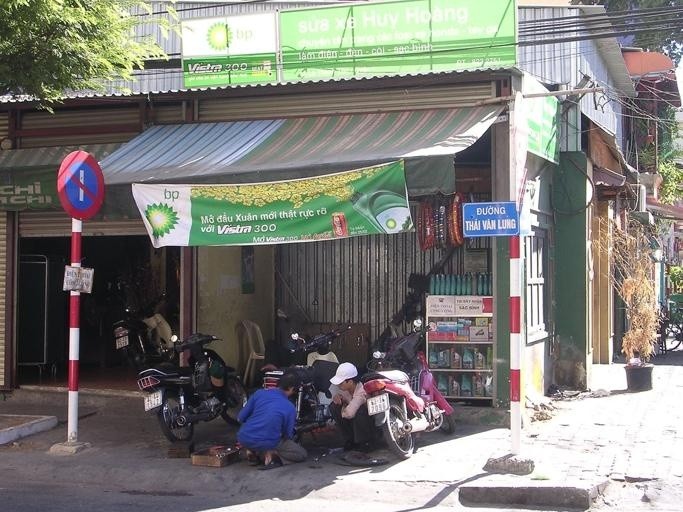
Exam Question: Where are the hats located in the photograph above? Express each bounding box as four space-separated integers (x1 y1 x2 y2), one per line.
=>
329 361 358 385
207 359 225 387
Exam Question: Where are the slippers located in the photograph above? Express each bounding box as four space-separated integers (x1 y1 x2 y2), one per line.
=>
247 460 259 466
257 460 280 470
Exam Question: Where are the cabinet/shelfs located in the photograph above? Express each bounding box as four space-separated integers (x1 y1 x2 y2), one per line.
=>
424 293 497 409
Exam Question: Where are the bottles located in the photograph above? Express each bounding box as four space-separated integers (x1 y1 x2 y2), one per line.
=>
429 272 493 295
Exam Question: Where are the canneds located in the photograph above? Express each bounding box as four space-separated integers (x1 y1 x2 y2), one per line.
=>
332 213 347 239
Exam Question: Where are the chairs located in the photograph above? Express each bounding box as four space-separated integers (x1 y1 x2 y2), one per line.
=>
239 318 268 384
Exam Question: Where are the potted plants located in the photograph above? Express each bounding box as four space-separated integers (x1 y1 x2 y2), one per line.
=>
592 215 664 391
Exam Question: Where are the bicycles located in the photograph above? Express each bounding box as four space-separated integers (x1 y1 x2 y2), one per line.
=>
656 300 683 356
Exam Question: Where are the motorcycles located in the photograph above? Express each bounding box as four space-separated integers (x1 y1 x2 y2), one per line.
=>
260 322 354 446
111 291 174 371
136 333 250 446
360 319 455 459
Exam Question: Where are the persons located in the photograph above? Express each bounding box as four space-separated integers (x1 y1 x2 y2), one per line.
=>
236 372 308 470
328 363 383 453
